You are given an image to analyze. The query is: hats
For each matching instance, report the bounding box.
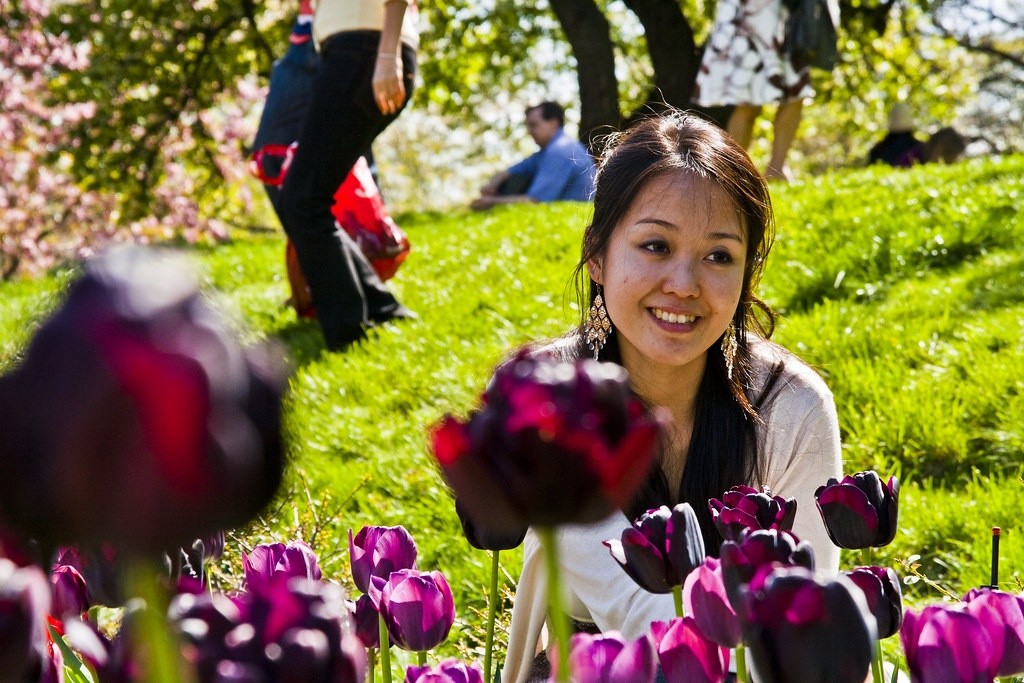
[889,104,913,131]
[289,0,314,45]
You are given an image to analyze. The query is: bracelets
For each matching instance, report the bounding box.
[377,52,401,59]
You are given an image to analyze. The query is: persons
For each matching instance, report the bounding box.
[486,111,908,682]
[691,0,811,184]
[253,0,421,352]
[466,100,597,212]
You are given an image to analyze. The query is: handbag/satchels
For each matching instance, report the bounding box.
[249,142,410,318]
[790,0,844,73]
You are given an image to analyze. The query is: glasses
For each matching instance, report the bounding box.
[529,117,550,130]
